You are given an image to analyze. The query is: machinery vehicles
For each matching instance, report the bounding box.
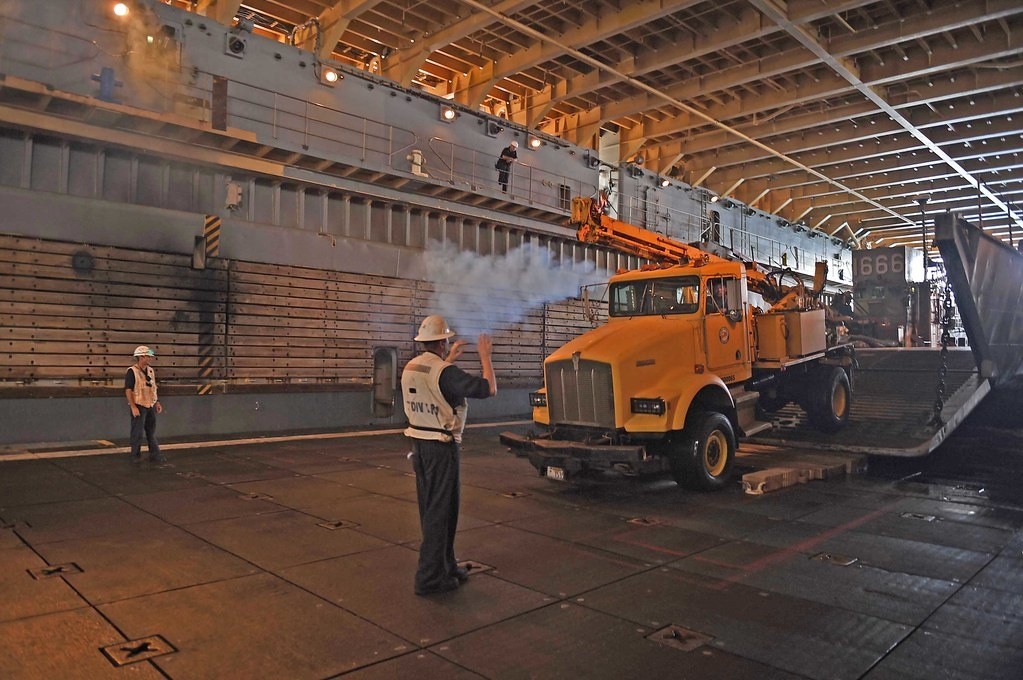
[499,173,858,493]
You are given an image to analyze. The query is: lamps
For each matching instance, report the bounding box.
[831,238,842,247]
[777,217,790,228]
[526,133,541,151]
[746,207,756,218]
[586,155,601,170]
[724,197,736,211]
[484,119,504,139]
[224,32,247,59]
[106,0,135,21]
[809,230,818,239]
[707,191,718,203]
[629,165,642,179]
[794,223,804,234]
[842,241,852,252]
[440,103,455,123]
[318,63,338,88]
[657,173,669,189]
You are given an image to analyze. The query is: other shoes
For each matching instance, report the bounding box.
[150,454,167,463]
[130,457,142,465]
[450,566,468,580]
[415,577,459,594]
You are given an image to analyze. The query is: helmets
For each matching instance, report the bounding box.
[134,346,154,356]
[511,141,518,149]
[413,315,455,342]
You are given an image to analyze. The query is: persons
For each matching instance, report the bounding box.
[401,314,497,596]
[125,345,167,465]
[496,140,518,194]
[713,284,727,310]
